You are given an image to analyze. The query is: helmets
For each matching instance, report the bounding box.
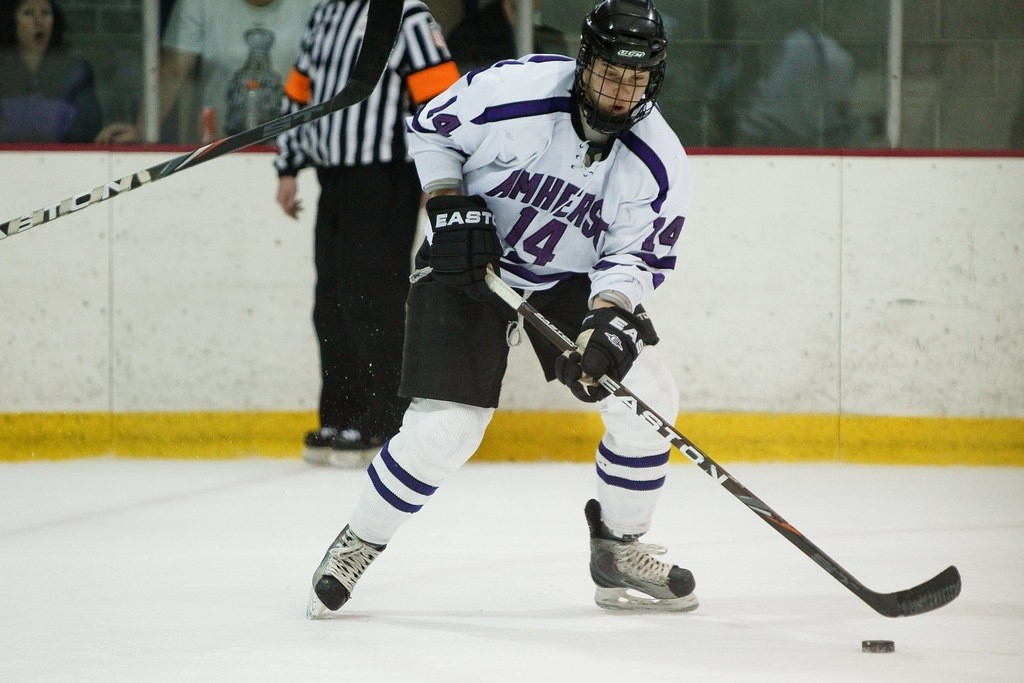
[575,0,669,135]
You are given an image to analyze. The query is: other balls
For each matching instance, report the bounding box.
[861,639,896,654]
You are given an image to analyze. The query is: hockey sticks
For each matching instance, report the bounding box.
[0,1,407,246]
[485,264,964,620]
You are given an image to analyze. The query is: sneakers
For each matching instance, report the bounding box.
[307,523,387,621]
[584,499,699,613]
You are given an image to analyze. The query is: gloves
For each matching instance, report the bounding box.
[555,303,659,404]
[426,195,503,301]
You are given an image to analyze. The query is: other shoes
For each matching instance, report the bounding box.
[303,424,384,467]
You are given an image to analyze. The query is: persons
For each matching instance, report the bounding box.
[305,0,698,621]
[275,0,460,465]
[0,0,136,143]
[444,0,857,143]
[95,0,318,147]
